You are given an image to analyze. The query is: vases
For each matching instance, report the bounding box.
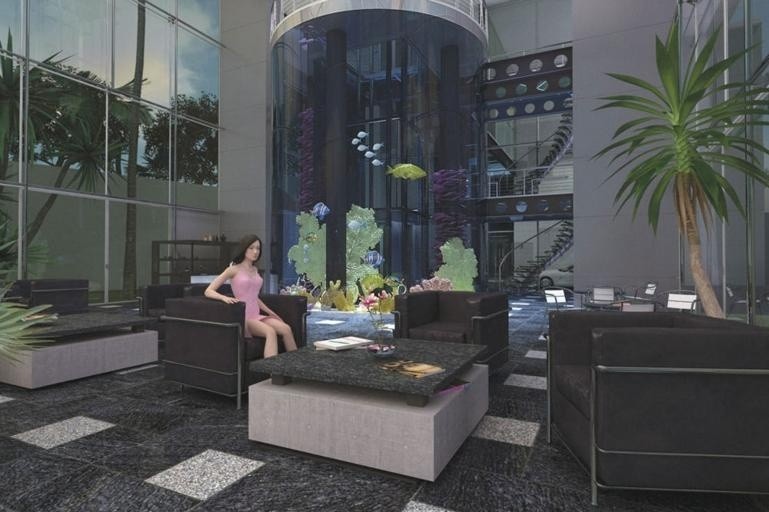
[366,320,398,357]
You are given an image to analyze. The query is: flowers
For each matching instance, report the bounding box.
[356,290,397,347]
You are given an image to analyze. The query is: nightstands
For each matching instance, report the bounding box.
[0,313,160,389]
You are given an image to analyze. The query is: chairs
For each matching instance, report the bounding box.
[546,310,768,508]
[3,279,89,315]
[542,281,700,314]
[136,283,312,410]
[392,291,510,378]
